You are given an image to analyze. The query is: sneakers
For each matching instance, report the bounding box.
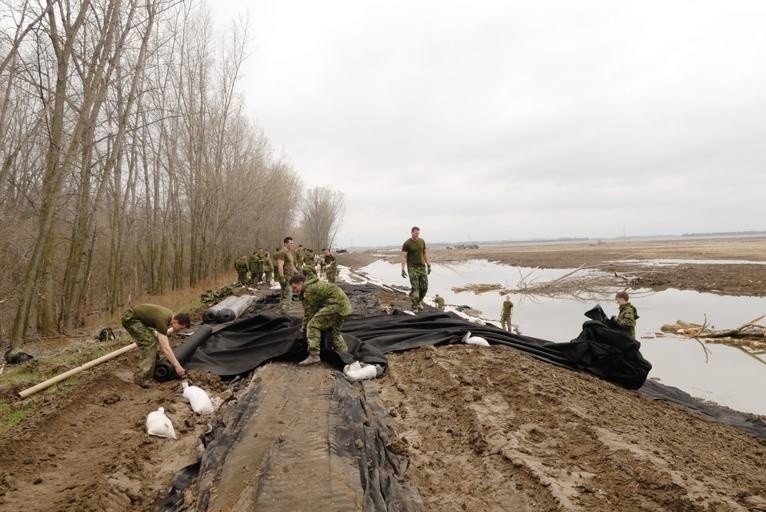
[413,304,424,314]
[137,379,160,387]
[298,354,321,367]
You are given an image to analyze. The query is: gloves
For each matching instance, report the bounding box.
[279,276,286,285]
[401,270,407,278]
[427,264,431,275]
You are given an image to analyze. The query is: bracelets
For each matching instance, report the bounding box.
[175,364,180,368]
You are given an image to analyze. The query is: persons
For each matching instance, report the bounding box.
[289,275,353,366]
[275,236,300,315]
[233,244,336,285]
[609,291,639,339]
[401,225,431,313]
[434,293,445,311]
[499,294,513,333]
[120,303,190,388]
